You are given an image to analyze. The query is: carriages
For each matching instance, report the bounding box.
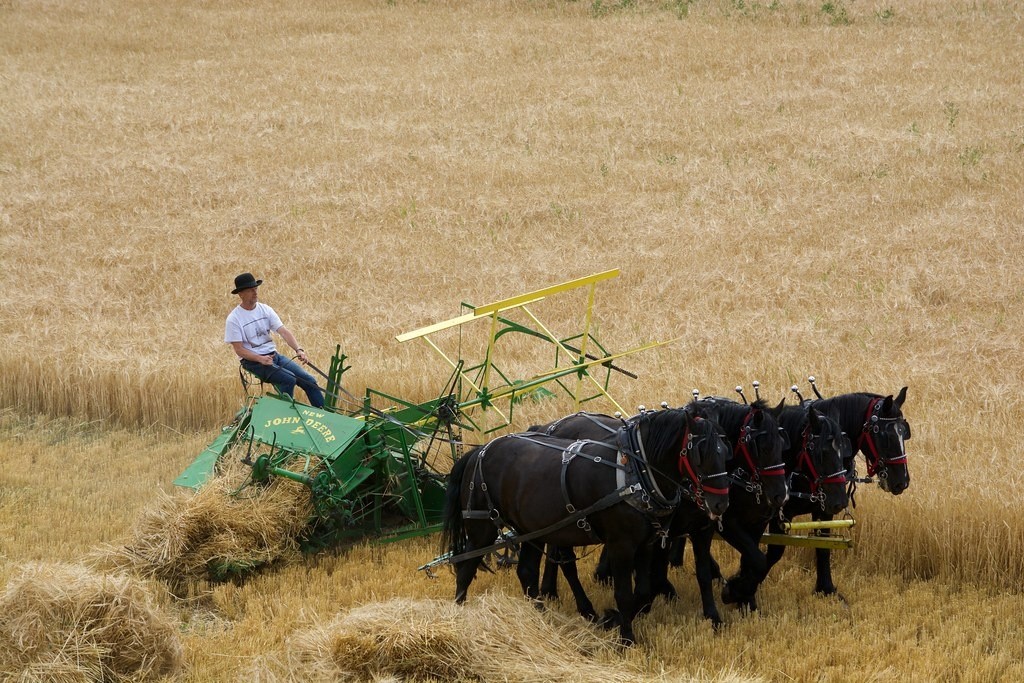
[173,268,912,651]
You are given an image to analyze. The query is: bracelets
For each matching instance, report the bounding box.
[295,348,304,353]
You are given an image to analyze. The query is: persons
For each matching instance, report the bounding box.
[225,273,325,409]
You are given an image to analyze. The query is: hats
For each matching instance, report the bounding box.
[231,273,262,294]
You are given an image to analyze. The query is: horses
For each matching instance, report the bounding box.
[437,386,912,647]
